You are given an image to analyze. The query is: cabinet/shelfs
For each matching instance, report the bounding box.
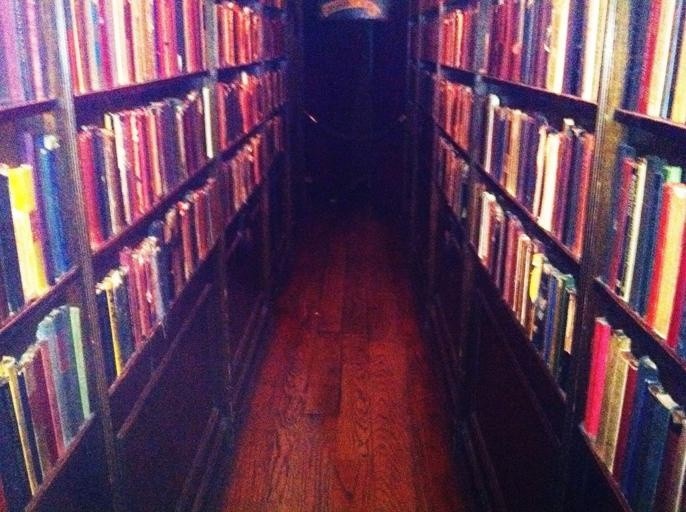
[0,0,298,512]
[405,1,686,512]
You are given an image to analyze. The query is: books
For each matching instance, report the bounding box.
[0,0,93,512]
[585,0,686,512]
[211,0,286,225]
[62,0,222,392]
[405,0,476,350]
[477,2,608,388]
